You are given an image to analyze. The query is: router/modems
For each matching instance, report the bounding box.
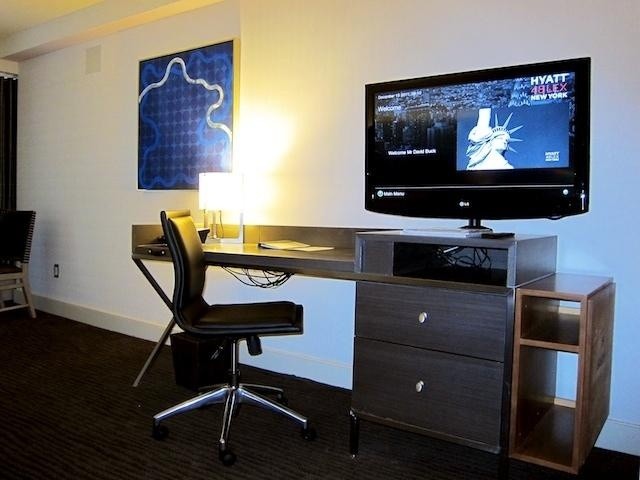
[203,209,245,244]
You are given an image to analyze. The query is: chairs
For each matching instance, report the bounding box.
[0,210,39,319]
[149,208,318,467]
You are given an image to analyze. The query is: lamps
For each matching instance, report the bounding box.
[199,171,250,242]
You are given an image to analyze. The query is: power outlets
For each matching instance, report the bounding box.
[53,263,59,278]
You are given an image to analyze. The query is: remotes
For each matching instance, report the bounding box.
[480,232,515,240]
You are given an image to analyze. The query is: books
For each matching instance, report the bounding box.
[258,239,336,253]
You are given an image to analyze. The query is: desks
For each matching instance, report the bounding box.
[132,224,403,397]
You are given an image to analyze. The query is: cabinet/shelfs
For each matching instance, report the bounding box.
[351,231,556,472]
[512,272,615,474]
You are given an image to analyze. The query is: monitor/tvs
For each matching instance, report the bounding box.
[364,56,592,237]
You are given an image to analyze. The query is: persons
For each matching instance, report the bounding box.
[464,108,516,171]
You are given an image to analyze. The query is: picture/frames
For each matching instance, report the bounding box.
[137,37,238,190]
